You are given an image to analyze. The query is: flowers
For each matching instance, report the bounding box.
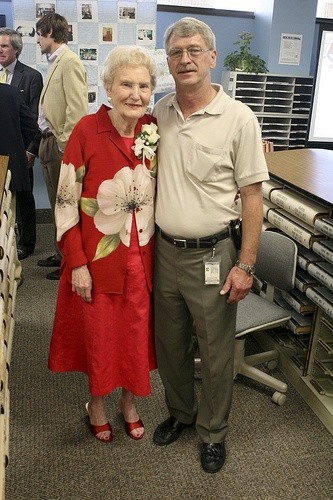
[130,122,161,173]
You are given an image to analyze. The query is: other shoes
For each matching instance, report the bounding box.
[16,245,33,260]
[202,442,226,473]
[46,269,61,280]
[37,254,61,267]
[153,416,187,446]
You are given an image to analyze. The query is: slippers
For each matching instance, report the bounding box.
[121,409,145,440]
[85,401,113,443]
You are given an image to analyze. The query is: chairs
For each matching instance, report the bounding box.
[192,231,302,409]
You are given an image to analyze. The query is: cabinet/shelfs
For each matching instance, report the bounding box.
[221,70,333,439]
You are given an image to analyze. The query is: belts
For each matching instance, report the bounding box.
[155,222,232,250]
[40,132,53,139]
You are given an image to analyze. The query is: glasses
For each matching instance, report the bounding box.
[166,46,210,58]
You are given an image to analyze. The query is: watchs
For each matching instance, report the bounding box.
[235,260,256,274]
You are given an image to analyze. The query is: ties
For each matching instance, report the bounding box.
[0,67,8,83]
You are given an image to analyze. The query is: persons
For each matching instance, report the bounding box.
[152,17,270,473]
[0,28,44,261]
[36,14,88,280]
[48,44,160,442]
